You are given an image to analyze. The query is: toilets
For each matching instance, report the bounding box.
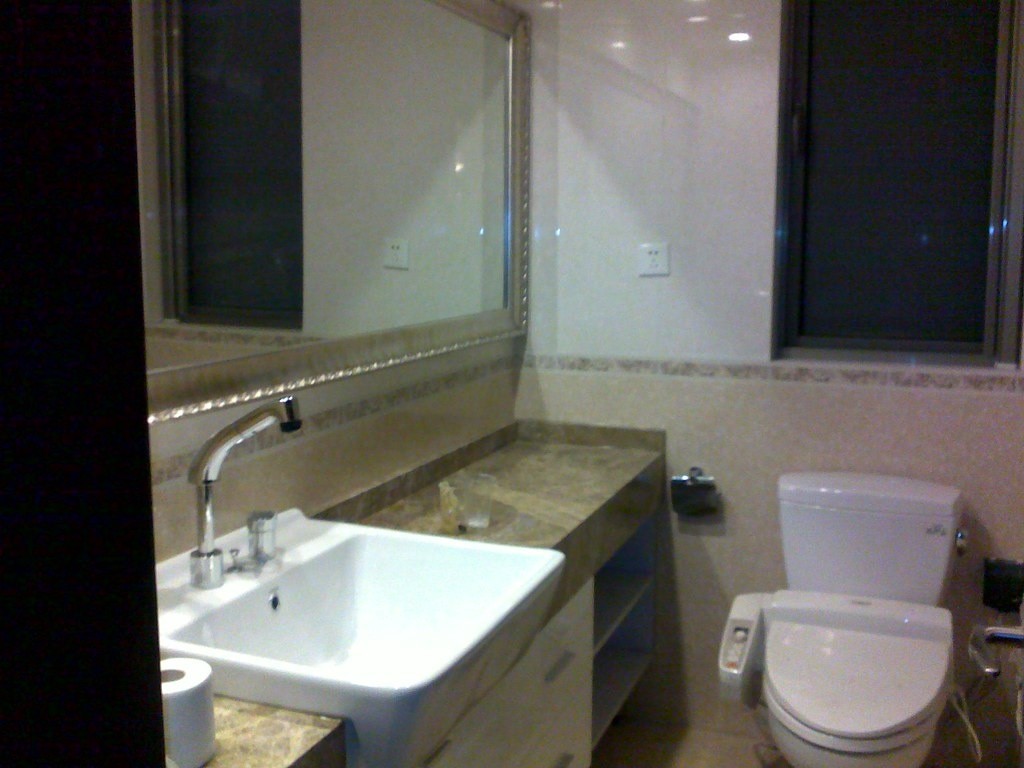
[719,470,972,768]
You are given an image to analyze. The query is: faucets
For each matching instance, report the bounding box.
[185,395,305,589]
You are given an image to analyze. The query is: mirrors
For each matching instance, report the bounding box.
[129,0,535,422]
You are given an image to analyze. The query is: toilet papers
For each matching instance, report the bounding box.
[157,656,218,768]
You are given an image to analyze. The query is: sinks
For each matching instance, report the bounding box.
[165,526,564,768]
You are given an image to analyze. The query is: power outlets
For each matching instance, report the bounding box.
[381,236,409,270]
[637,240,670,278]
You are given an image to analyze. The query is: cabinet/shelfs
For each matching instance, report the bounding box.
[593,447,657,748]
[415,573,593,768]
